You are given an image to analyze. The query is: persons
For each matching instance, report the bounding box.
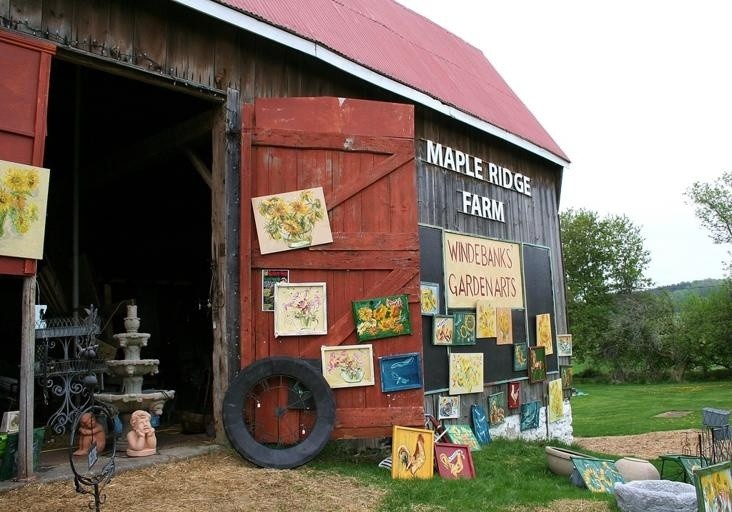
[126,410,158,456]
[73,413,106,456]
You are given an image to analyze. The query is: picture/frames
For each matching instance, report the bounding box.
[570,453,732,512]
[388,395,492,484]
[432,302,496,347]
[273,282,328,336]
[487,306,574,432]
[319,344,376,389]
[378,351,424,393]
[419,280,441,318]
